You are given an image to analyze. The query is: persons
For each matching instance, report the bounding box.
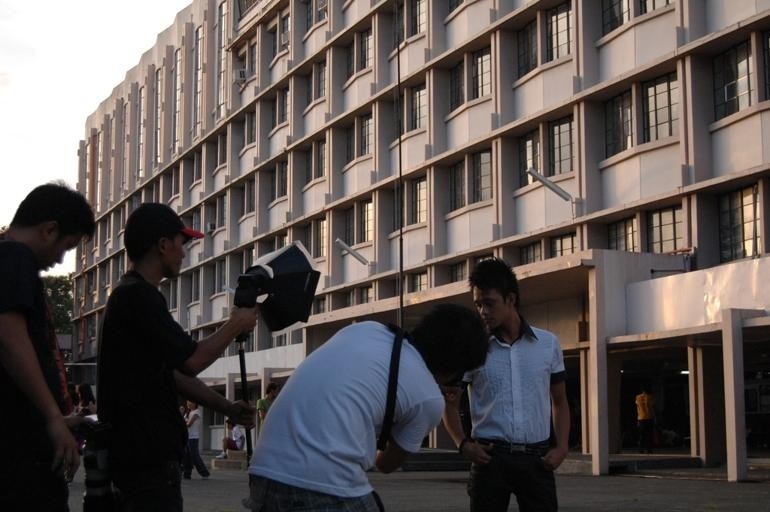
[82,200,259,511]
[78,383,96,415]
[634,384,658,454]
[67,382,79,416]
[442,257,573,512]
[257,381,284,431]
[0,181,96,511]
[216,418,245,460]
[183,400,210,480]
[241,302,493,511]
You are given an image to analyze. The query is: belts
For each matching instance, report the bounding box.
[482,442,545,454]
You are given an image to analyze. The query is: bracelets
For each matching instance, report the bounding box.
[459,436,471,453]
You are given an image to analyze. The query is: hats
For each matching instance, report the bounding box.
[129,203,205,245]
